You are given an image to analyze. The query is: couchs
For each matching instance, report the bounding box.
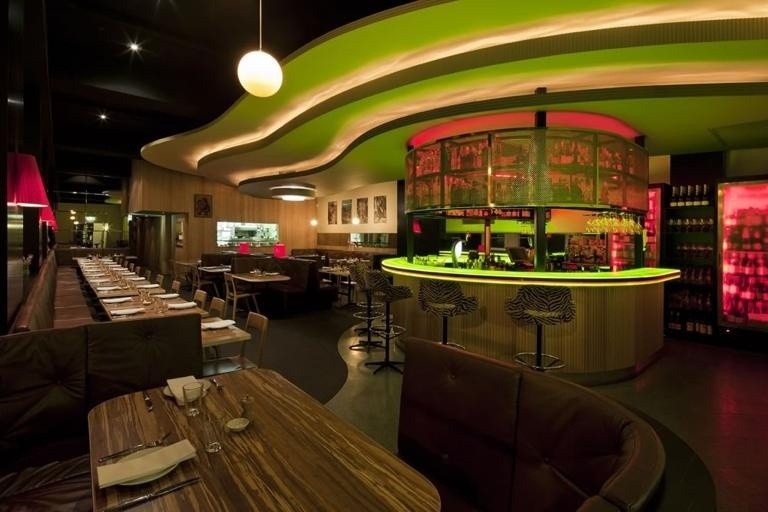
[234,256,311,313]
[390,336,720,509]
[0,244,211,475]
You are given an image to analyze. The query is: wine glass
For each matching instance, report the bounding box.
[582,212,644,235]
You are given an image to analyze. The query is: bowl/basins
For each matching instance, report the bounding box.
[226,417,250,432]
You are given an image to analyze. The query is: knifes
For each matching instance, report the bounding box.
[141,389,154,412]
[94,476,202,512]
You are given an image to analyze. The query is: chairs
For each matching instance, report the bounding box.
[362,271,413,375]
[347,264,388,354]
[290,249,376,310]
[354,288,384,338]
[502,283,577,373]
[413,277,481,351]
[3,247,269,380]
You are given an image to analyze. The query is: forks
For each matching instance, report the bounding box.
[98,431,176,464]
[207,375,225,394]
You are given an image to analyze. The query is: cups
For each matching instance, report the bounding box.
[254,267,261,276]
[200,409,225,455]
[83,254,168,315]
[182,382,204,417]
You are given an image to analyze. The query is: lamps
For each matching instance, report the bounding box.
[238,241,250,256]
[232,1,284,100]
[7,150,61,233]
[272,243,285,258]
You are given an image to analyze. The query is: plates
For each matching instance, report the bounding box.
[171,302,198,311]
[163,378,212,398]
[200,326,225,330]
[117,446,179,486]
[168,293,178,300]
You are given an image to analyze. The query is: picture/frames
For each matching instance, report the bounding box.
[193,192,214,219]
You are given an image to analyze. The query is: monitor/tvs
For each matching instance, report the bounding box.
[507,247,527,263]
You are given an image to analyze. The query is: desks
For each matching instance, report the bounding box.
[82,364,442,512]
[233,270,291,317]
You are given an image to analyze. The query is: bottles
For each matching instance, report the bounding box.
[333,263,346,272]
[238,395,255,424]
[669,243,713,282]
[666,182,712,208]
[666,218,714,232]
[669,292,714,336]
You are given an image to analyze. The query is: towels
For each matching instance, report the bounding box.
[165,373,209,406]
[90,438,204,488]
